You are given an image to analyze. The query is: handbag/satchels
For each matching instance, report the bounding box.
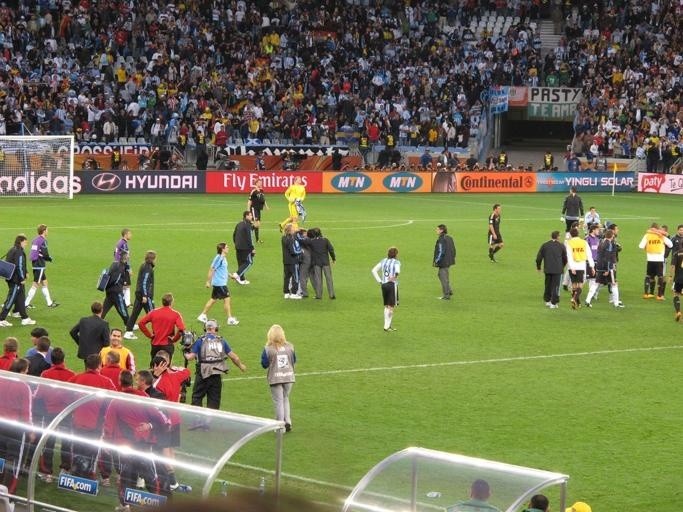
[0,247,17,281]
[96,268,110,292]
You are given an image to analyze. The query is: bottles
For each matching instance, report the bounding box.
[221,481,228,498]
[259,477,265,496]
[424,491,442,499]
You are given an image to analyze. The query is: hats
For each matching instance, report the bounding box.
[204,319,220,327]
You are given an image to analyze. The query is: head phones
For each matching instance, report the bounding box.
[204,320,218,332]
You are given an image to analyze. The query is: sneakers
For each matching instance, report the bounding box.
[233,272,250,285]
[283,284,336,300]
[37,468,193,512]
[0,301,37,328]
[561,280,626,310]
[674,311,682,322]
[48,302,59,308]
[227,317,240,326]
[383,326,398,331]
[123,323,140,340]
[197,313,209,324]
[545,300,559,309]
[641,293,667,301]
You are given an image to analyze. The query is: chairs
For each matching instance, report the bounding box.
[443,8,600,49]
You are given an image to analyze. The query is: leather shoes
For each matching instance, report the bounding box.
[441,292,453,300]
